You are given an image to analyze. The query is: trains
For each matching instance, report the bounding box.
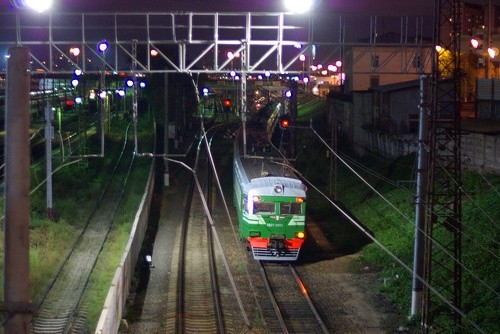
[231,101,307,263]
